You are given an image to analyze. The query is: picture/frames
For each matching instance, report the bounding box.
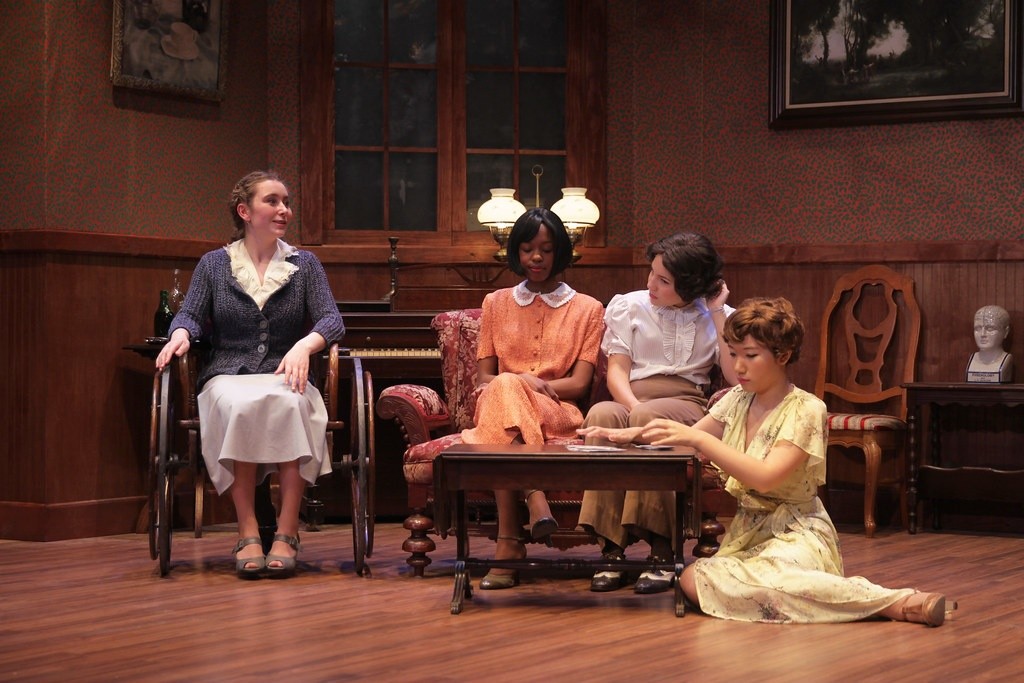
[109,0,230,105]
[769,0,1024,133]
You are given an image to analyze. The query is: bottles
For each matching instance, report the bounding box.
[154,290,174,339]
[169,269,186,314]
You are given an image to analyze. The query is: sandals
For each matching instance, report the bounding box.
[232,531,304,580]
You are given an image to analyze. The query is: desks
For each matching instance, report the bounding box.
[894,369,1024,533]
[434,441,693,617]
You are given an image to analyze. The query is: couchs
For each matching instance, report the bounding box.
[376,307,726,577]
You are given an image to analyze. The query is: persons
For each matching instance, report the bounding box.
[155,170,346,579]
[462,206,605,588]
[576,232,737,594]
[964,304,1014,385]
[576,296,958,626]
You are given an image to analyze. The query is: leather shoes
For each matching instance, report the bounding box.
[634,554,676,593]
[591,548,626,589]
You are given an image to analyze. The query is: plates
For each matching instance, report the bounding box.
[144,336,168,345]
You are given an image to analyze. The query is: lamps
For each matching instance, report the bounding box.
[477,187,527,263]
[550,188,599,260]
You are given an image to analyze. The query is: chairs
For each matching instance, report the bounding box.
[813,264,923,526]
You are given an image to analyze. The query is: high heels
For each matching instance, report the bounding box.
[904,586,958,627]
[525,488,559,546]
[479,535,528,589]
